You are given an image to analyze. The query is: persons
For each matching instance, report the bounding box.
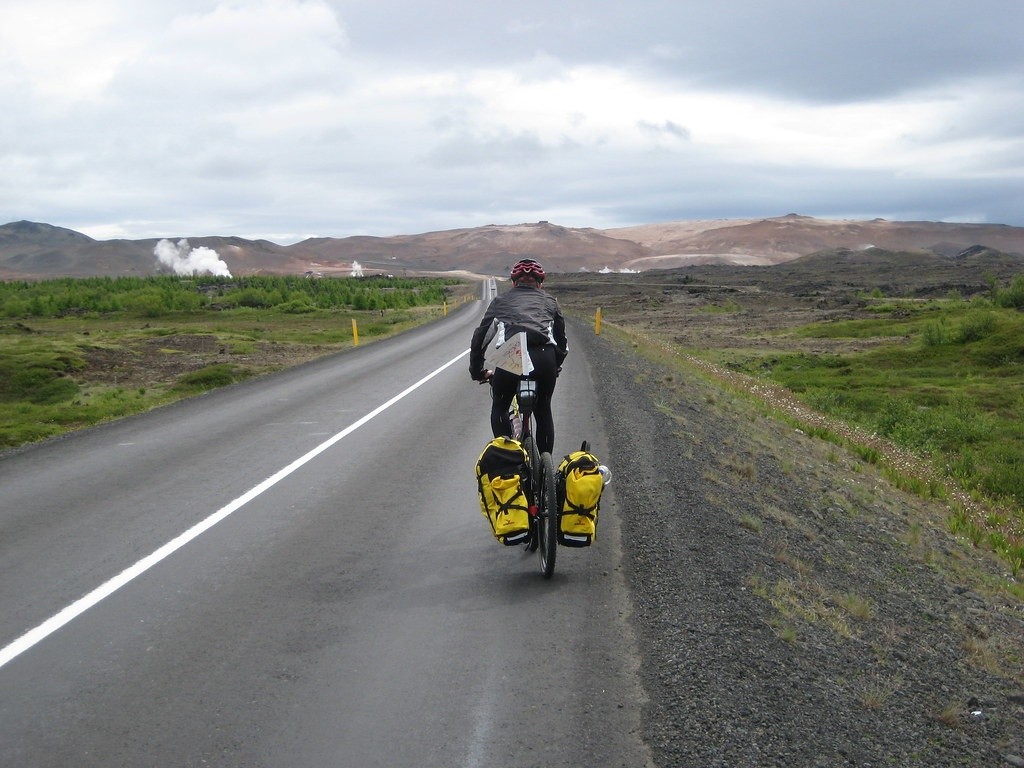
[468,259,568,457]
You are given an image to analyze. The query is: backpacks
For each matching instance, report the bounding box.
[556,440,607,549]
[477,438,533,544]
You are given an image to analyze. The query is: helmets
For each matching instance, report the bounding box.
[510,258,545,286]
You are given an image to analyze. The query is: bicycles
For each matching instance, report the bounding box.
[478,367,565,576]
